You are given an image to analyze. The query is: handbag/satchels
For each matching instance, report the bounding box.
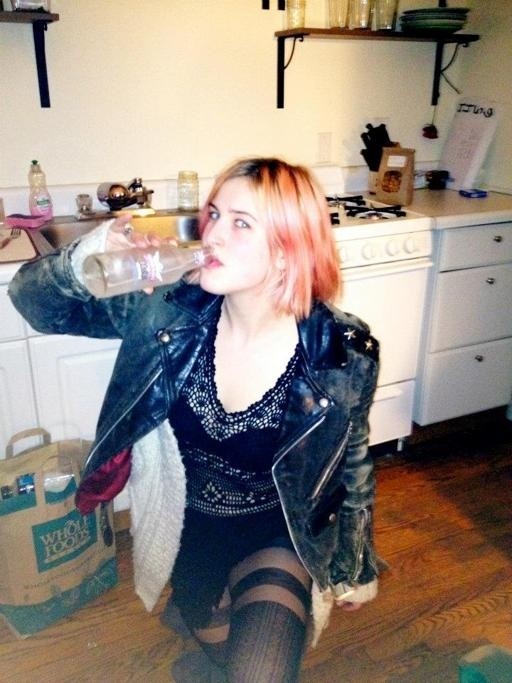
[1,427,119,641]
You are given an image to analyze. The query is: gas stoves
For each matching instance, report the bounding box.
[321,191,430,229]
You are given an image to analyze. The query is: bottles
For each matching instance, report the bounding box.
[81,246,212,299]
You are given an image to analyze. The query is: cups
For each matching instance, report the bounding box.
[328,0,399,30]
[177,169,200,212]
[287,0,306,30]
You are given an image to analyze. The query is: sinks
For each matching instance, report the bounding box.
[33,214,202,252]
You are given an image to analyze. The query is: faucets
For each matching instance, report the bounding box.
[99,184,148,214]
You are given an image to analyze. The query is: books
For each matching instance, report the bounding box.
[438,96,499,190]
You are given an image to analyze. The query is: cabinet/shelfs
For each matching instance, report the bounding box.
[0,262,42,464]
[411,218,512,428]
[24,262,138,532]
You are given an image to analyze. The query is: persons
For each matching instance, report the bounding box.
[7,156,380,682]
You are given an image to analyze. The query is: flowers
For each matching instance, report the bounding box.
[422,94,438,138]
